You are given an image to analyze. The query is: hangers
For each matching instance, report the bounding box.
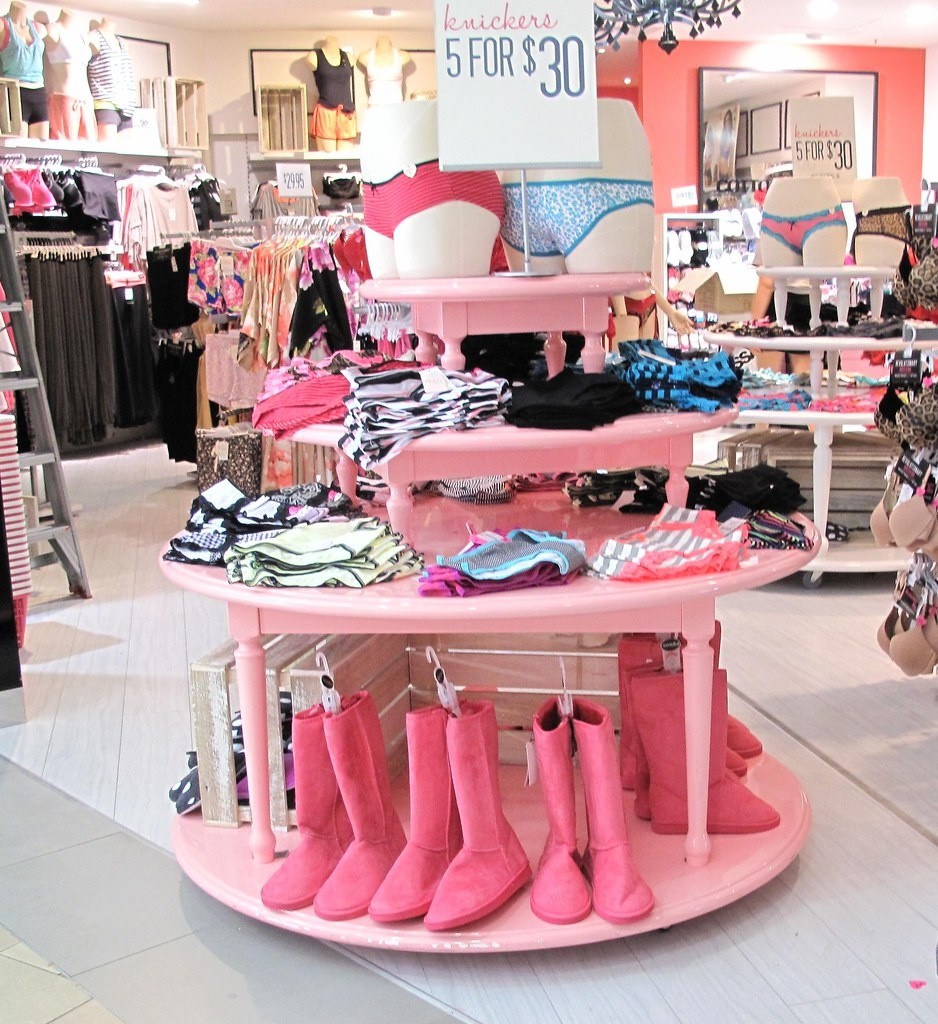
[886,317,932,371]
[268,163,361,187]
[2,150,99,169]
[12,230,101,260]
[115,164,226,191]
[910,177,936,213]
[148,214,364,257]
[351,303,413,342]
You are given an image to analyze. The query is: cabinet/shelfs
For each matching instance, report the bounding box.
[652,212,745,464]
[135,274,812,953]
[706,267,938,589]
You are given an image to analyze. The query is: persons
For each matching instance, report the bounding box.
[760,175,848,268]
[307,36,356,152]
[46,9,94,141]
[0,0,49,139]
[612,283,694,354]
[853,176,911,268]
[501,99,655,274]
[751,279,814,374]
[88,18,134,143]
[359,37,412,106]
[360,99,504,279]
[718,110,735,181]
[705,128,713,186]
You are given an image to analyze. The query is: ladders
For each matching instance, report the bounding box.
[0,192,92,600]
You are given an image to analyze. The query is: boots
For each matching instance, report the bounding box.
[261,696,356,911]
[368,696,464,922]
[619,619,762,821]
[312,690,407,921]
[631,669,781,834]
[422,699,532,931]
[569,698,654,924]
[533,695,593,925]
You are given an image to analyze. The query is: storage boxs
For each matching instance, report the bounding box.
[0,76,23,137]
[187,625,413,839]
[406,634,624,763]
[255,84,310,154]
[716,424,905,533]
[671,267,758,314]
[140,77,210,153]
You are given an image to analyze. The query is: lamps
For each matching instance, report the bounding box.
[594,0,741,52]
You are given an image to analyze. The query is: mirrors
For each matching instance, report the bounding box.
[697,64,880,212]
